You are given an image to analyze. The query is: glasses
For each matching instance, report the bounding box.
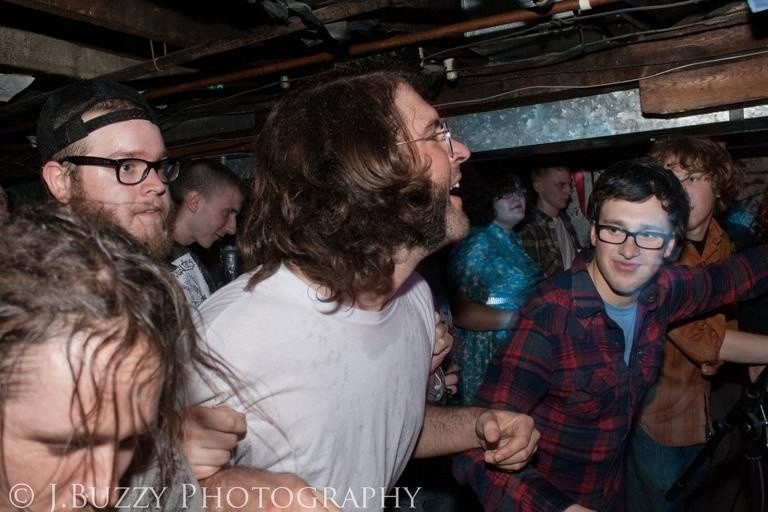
[393,117,454,159]
[57,153,183,187]
[594,217,678,252]
[497,184,530,200]
